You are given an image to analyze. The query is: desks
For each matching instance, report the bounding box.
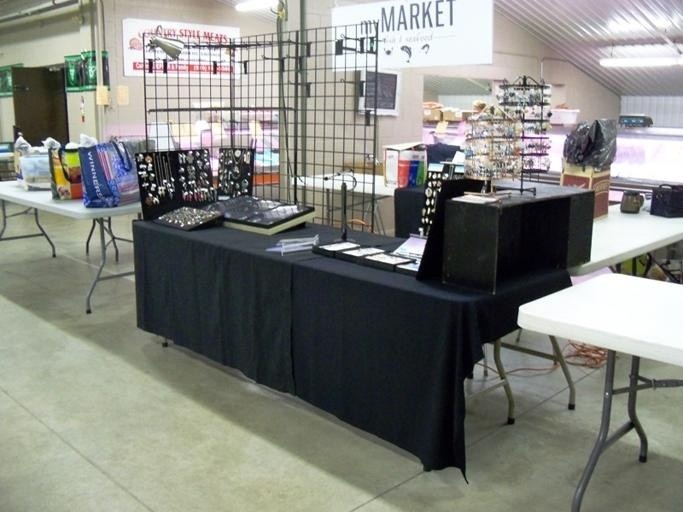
[0,181,143,314]
[568,199,683,277]
[133,210,574,482]
[516,274,682,511]
[292,172,395,238]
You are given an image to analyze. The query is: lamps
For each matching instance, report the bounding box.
[148,35,185,59]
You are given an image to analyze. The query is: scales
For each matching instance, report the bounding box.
[619,115,653,127]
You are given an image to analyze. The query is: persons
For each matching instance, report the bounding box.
[472,100,486,112]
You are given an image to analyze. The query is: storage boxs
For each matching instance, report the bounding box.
[382,143,427,188]
[422,101,579,123]
[560,158,610,218]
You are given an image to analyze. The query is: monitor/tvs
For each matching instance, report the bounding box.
[358,68,402,117]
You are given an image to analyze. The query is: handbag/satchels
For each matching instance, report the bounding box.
[48,145,84,200]
[79,140,155,207]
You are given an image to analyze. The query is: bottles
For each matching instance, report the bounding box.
[399,155,420,188]
